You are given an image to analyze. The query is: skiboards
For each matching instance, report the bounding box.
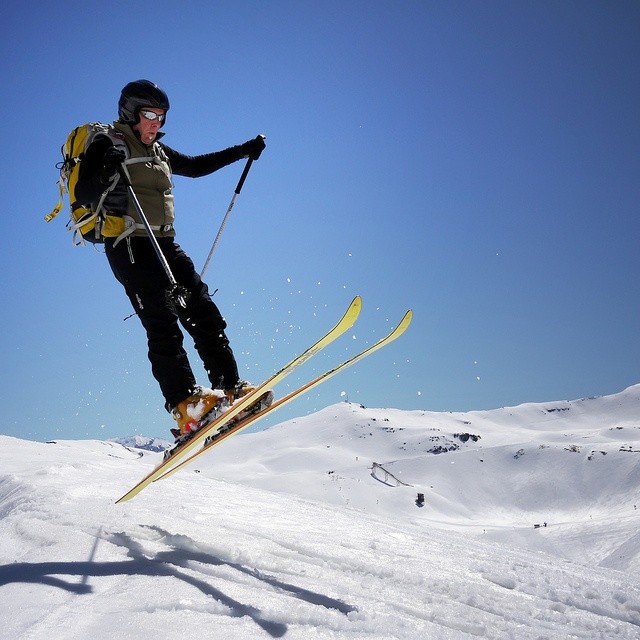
[113,295,413,504]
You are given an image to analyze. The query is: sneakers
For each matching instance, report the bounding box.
[216,385,272,432]
[175,390,232,437]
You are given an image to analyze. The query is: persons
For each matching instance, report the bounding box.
[74,80,272,446]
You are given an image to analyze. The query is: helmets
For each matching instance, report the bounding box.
[119,80,169,125]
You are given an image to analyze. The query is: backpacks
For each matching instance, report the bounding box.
[44,122,119,244]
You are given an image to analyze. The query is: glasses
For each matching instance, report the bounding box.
[139,110,165,121]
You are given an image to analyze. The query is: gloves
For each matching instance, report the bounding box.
[101,147,124,177]
[244,137,266,159]
